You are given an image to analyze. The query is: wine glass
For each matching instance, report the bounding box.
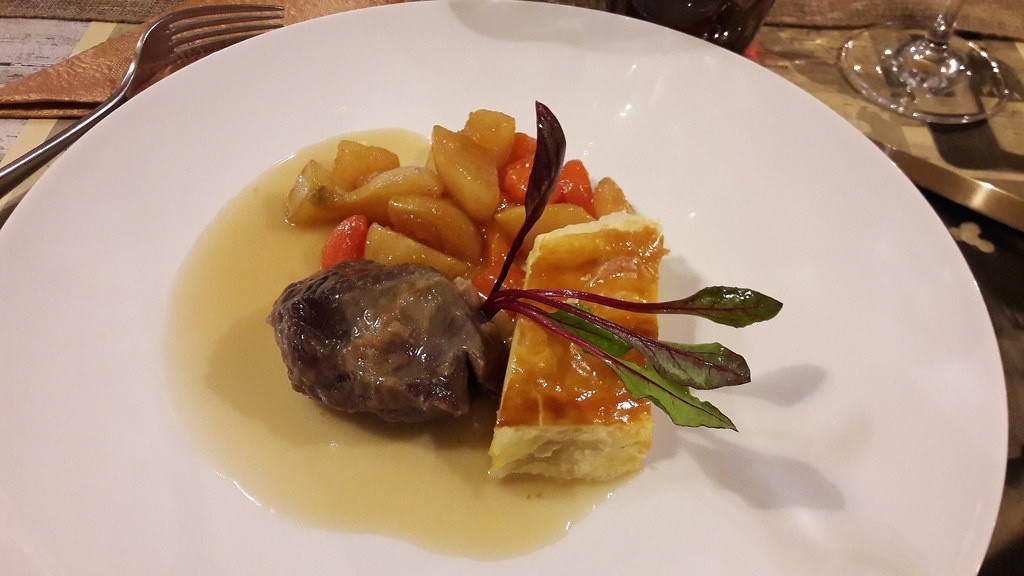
[836,0,1014,129]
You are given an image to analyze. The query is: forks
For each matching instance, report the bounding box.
[1,3,285,203]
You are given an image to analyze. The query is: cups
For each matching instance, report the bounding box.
[602,1,775,58]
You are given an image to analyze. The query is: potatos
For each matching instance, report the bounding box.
[282,104,637,327]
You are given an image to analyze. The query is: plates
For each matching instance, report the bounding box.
[0,0,1012,574]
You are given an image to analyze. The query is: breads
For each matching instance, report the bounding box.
[486,210,670,478]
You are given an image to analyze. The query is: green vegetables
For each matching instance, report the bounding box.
[477,99,783,432]
